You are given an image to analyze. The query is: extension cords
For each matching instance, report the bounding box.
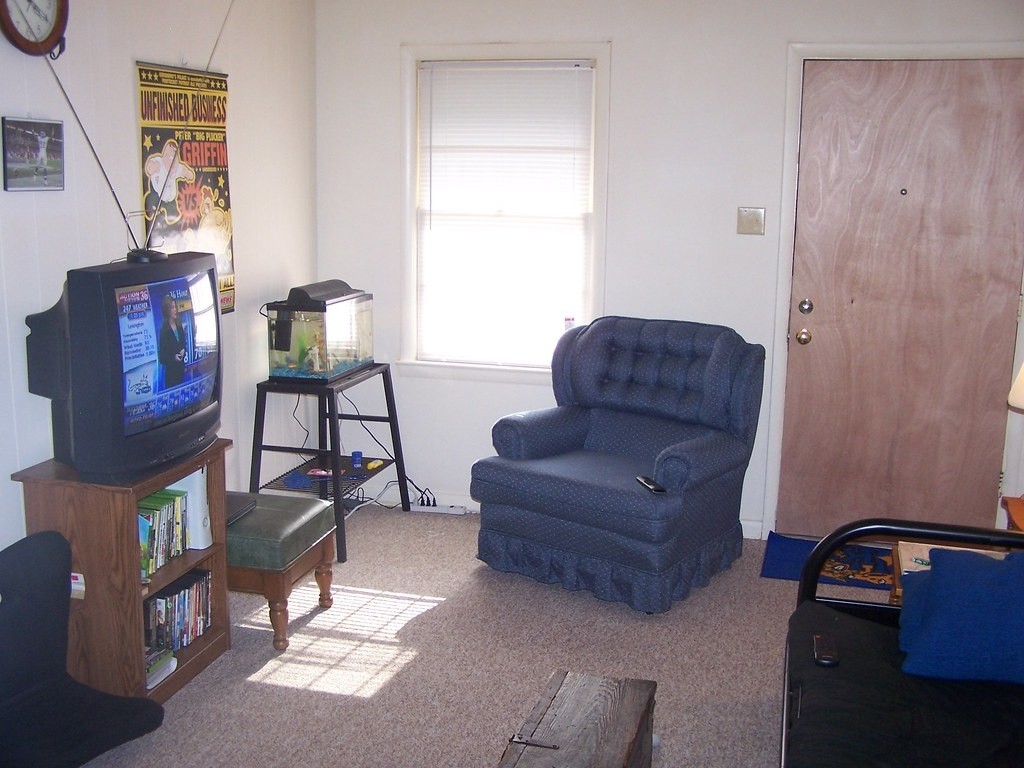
[410,503,467,515]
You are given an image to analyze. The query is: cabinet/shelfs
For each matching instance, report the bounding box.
[250,364,411,562]
[10,438,234,703]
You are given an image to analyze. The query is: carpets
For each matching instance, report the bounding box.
[761,530,894,590]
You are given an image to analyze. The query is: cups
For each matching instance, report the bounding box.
[352,451,362,468]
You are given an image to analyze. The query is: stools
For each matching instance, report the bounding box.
[225,491,337,649]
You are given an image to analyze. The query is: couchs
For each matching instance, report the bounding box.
[470,316,765,613]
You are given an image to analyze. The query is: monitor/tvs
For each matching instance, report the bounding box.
[26,251,224,475]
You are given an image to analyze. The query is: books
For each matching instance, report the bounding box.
[137,488,190,578]
[143,568,212,689]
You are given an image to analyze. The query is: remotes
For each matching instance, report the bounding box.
[812,632,841,666]
[636,475,666,494]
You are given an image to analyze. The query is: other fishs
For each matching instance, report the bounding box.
[271,309,373,373]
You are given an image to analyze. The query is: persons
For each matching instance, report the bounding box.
[158,295,186,390]
[32,127,55,186]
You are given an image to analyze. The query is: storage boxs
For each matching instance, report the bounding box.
[497,669,658,767]
[259,280,373,384]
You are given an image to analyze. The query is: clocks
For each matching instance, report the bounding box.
[0,0,69,60]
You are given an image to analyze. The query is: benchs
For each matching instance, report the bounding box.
[780,518,1023,768]
